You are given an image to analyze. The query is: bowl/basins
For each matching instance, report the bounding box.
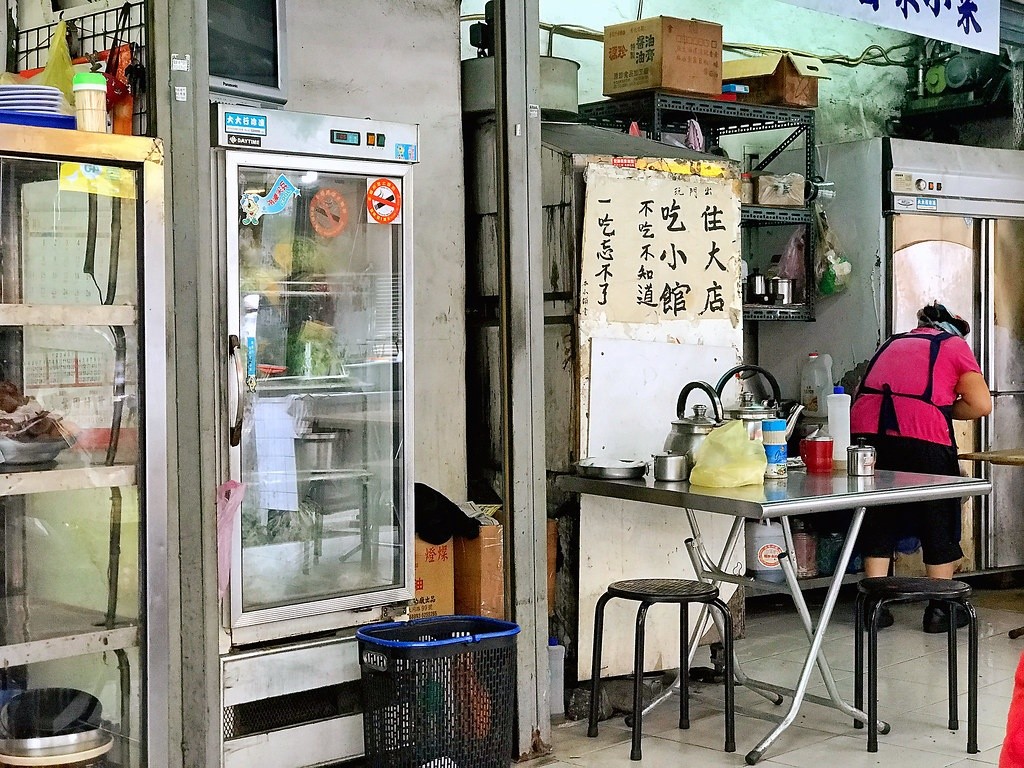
[0,435,76,462]
[294,426,348,476]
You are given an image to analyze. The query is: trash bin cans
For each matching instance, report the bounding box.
[356,615,521,768]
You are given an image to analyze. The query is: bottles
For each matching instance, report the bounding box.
[547,635,564,715]
[240,294,259,376]
[745,517,786,570]
[799,353,833,418]
[827,386,851,460]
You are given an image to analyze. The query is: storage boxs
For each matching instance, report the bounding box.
[722,52,832,109]
[602,15,723,98]
[382,534,454,622]
[453,525,504,620]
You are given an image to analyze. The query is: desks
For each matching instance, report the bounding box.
[958,448,1024,640]
[550,459,992,766]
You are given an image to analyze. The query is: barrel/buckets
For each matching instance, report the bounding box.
[0,688,114,768]
[792,519,818,577]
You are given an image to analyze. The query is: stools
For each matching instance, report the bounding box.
[854,577,978,754]
[587,579,735,761]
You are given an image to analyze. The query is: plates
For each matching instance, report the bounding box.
[0,85,64,114]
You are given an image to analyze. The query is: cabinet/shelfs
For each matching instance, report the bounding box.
[577,91,817,321]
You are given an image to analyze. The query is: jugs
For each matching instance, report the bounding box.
[663,381,724,478]
[716,365,805,441]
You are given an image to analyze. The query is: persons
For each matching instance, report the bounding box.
[850,302,994,631]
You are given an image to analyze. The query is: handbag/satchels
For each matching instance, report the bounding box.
[690,419,767,487]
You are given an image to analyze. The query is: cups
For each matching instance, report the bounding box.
[800,437,834,475]
[763,442,787,479]
[771,278,798,303]
[772,278,793,304]
[742,280,748,302]
[746,267,765,295]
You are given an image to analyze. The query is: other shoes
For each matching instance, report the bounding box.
[864,604,894,630]
[924,606,970,633]
